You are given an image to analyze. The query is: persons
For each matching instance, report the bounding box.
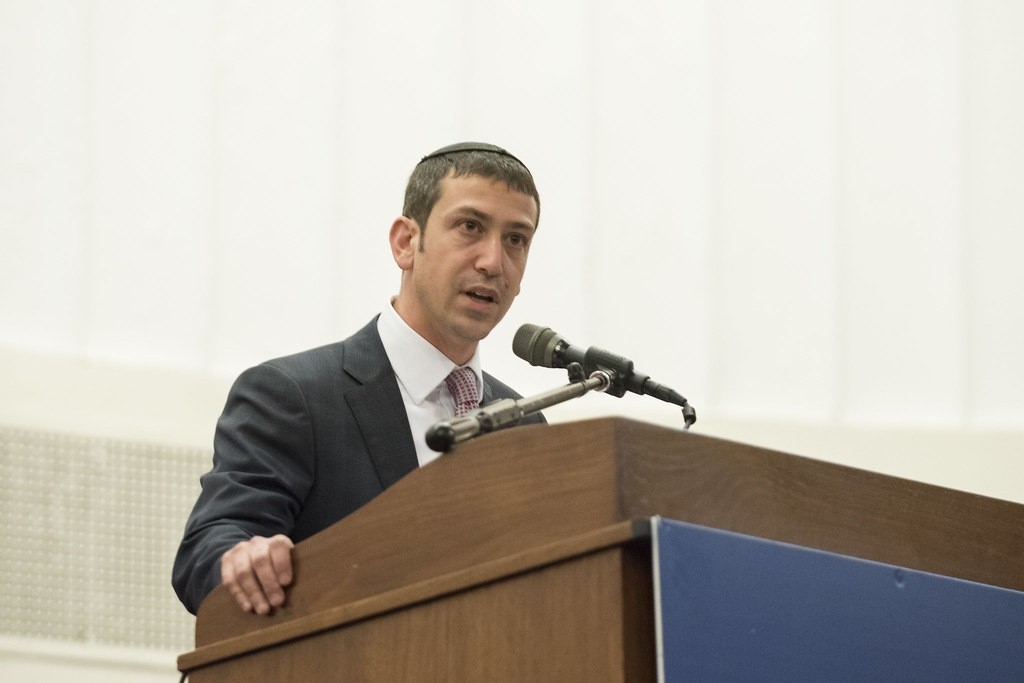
[170,141,549,616]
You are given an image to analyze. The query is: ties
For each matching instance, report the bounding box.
[445,368,479,418]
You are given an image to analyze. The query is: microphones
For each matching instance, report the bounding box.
[512,323,687,406]
